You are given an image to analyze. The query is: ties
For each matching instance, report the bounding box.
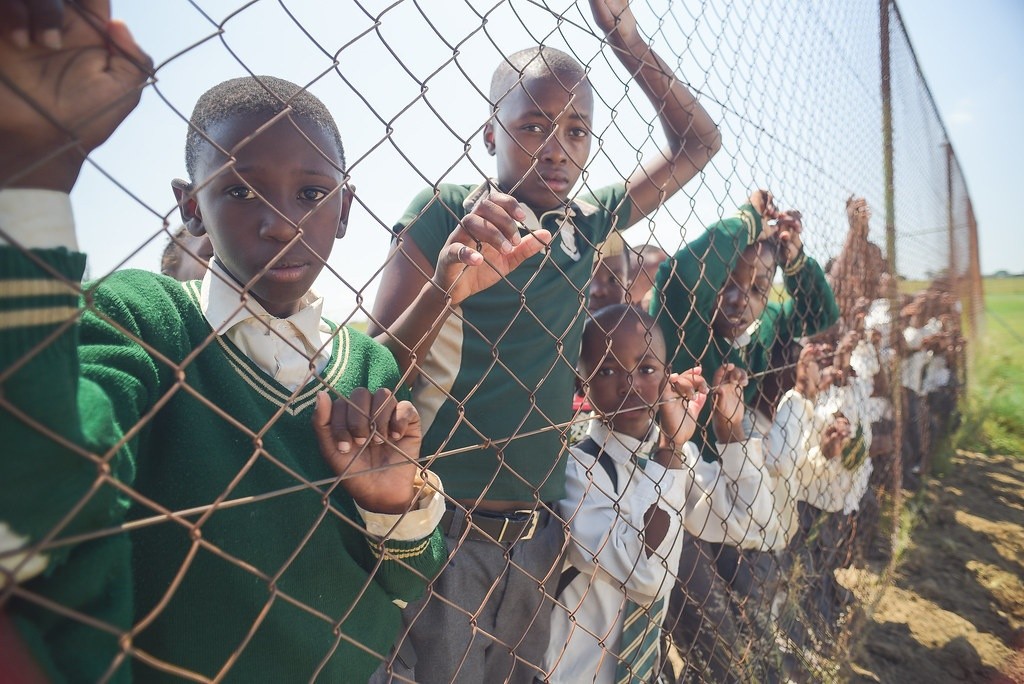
[615,443,673,684]
[841,412,869,471]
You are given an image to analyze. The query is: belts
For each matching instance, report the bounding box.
[440,503,552,543]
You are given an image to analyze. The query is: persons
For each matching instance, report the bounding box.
[160,0,968,684]
[0,0,446,684]
[368,1,723,684]
